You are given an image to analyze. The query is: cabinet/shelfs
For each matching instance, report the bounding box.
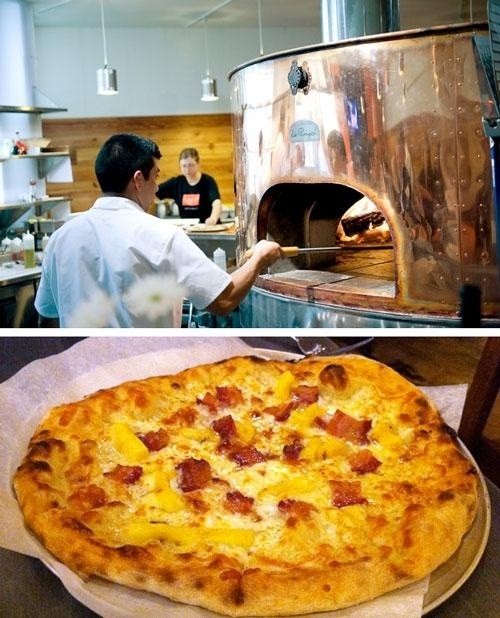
[0,107,72,212]
[0,262,60,328]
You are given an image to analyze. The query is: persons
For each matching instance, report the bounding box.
[33,131,286,328]
[390,132,439,237]
[150,146,225,226]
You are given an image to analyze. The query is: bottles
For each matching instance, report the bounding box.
[1,236,22,262]
[213,246,227,273]
[41,232,49,251]
[22,229,35,268]
[13,131,21,154]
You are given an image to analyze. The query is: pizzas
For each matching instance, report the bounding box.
[8,353,479,617]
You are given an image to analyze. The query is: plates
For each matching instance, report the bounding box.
[421,432,492,615]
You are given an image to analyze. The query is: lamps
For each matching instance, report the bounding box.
[201,18,219,101]
[97,1,117,96]
